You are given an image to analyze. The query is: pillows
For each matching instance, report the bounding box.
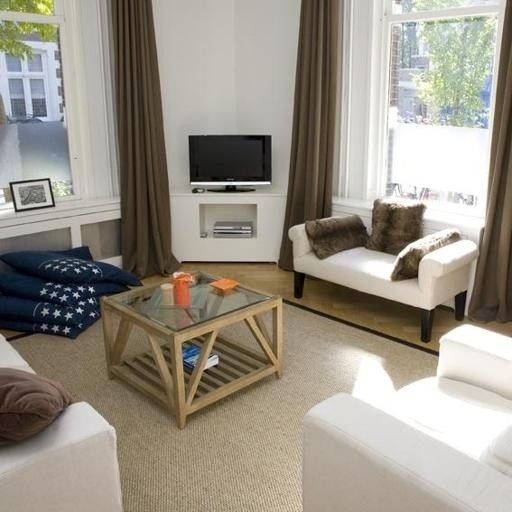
[0,366,75,445]
[366,198,426,254]
[302,214,369,260]
[0,245,146,340]
[384,226,461,283]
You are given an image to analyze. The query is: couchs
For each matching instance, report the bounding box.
[0,320,130,512]
[284,193,481,344]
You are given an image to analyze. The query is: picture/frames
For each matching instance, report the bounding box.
[10,177,56,213]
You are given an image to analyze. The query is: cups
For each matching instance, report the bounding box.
[172,272,192,308]
[162,284,174,306]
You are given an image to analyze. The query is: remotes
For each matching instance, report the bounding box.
[192,188,199,193]
[197,189,205,192]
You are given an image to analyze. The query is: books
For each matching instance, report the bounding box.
[182,345,219,371]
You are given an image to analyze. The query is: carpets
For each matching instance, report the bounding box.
[6,275,442,512]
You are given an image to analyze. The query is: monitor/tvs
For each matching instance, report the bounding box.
[189,134,272,193]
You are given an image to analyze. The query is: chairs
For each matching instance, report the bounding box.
[293,317,512,511]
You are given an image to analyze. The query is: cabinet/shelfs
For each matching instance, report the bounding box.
[167,188,293,268]
